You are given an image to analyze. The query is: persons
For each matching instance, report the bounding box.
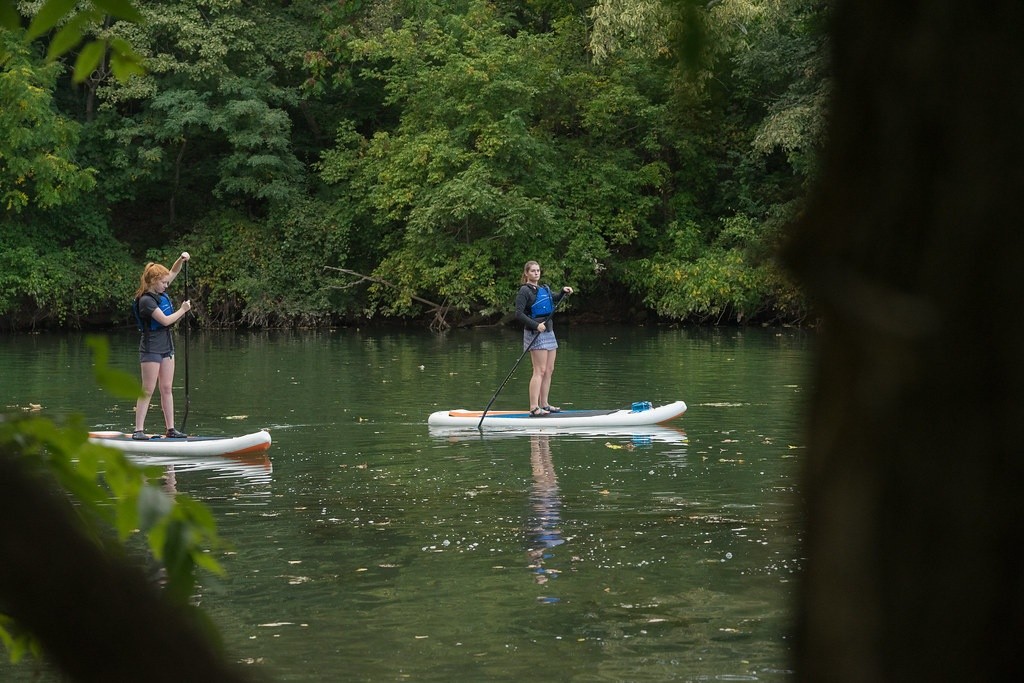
[132,252,191,440]
[515,261,573,417]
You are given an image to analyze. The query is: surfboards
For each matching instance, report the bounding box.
[86,428,273,457]
[427,401,688,429]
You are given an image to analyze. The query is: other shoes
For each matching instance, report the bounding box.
[166,428,187,438]
[132,430,149,440]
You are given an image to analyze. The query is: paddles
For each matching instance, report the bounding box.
[476,290,569,429]
[176,258,191,434]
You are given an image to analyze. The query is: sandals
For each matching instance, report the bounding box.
[529,407,551,417]
[542,405,560,413]
